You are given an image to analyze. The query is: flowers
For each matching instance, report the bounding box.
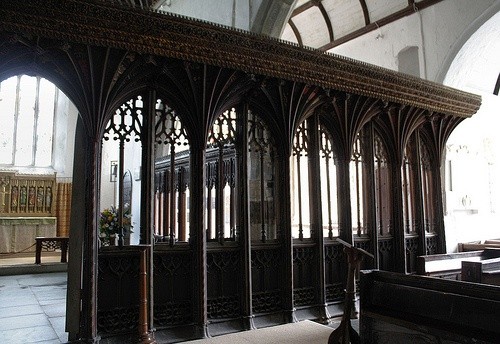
[100,204,136,239]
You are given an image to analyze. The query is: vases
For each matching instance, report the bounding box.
[100,236,117,249]
